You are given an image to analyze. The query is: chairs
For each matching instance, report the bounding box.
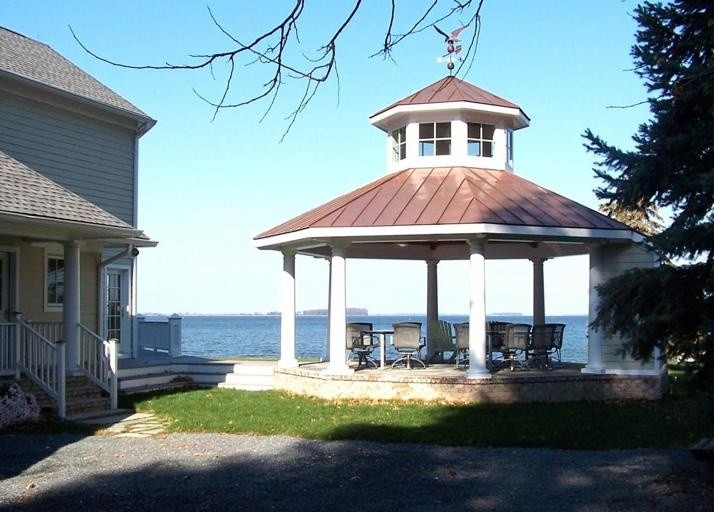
[344,319,566,372]
